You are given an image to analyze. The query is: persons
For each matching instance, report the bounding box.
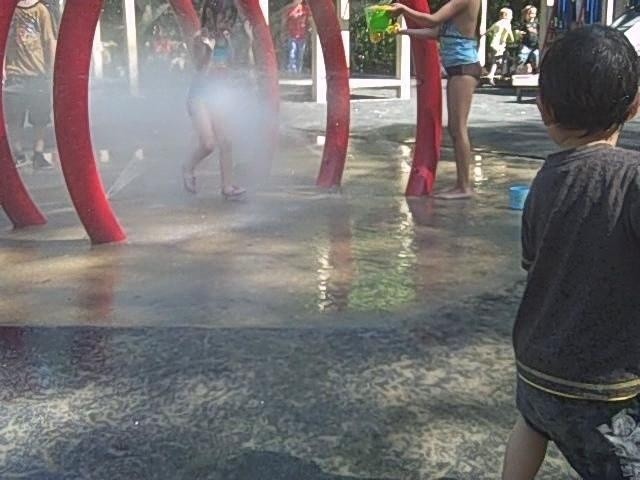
[182,0,248,197]
[384,0,484,200]
[480,7,515,86]
[2,0,54,168]
[27,1,235,78]
[279,1,312,80]
[501,22,640,479]
[513,3,541,73]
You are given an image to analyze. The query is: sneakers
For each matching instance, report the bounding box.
[221,183,246,196]
[13,150,33,167]
[181,161,198,193]
[33,152,53,170]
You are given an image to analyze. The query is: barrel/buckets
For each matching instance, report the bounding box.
[509,186,530,209]
[364,6,394,32]
